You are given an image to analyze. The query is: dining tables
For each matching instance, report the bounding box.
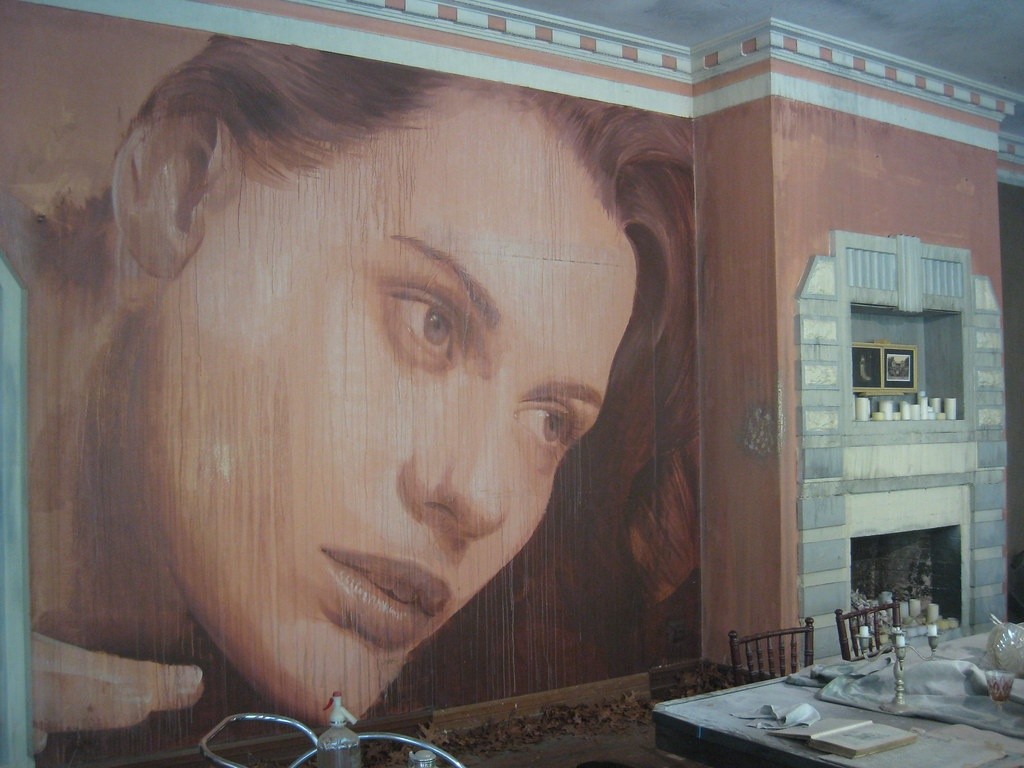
[652,620,1024,767]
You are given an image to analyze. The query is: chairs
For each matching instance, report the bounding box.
[728,617,814,686]
[834,596,901,661]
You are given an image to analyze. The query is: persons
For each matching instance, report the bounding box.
[27,2,704,768]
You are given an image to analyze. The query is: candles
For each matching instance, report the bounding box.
[856,390,957,420]
[859,592,960,649]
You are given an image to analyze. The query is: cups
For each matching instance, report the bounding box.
[985,670,1015,712]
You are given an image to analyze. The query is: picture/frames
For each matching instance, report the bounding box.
[852,342,918,396]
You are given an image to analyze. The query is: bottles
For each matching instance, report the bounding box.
[315,691,363,768]
